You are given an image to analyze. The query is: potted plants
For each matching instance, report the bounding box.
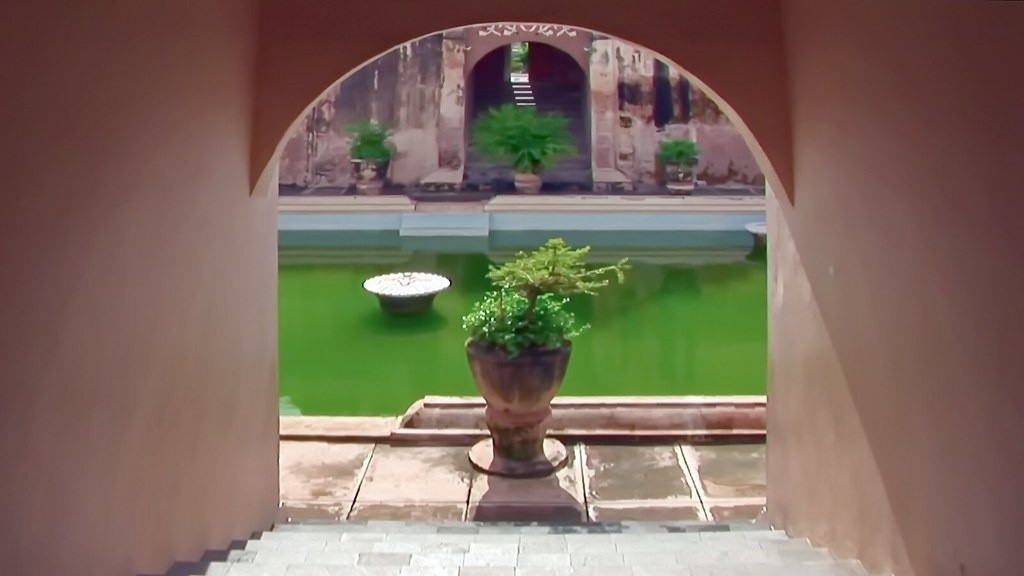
[658,140,699,183]
[343,121,398,191]
[462,237,636,478]
[471,101,578,194]
